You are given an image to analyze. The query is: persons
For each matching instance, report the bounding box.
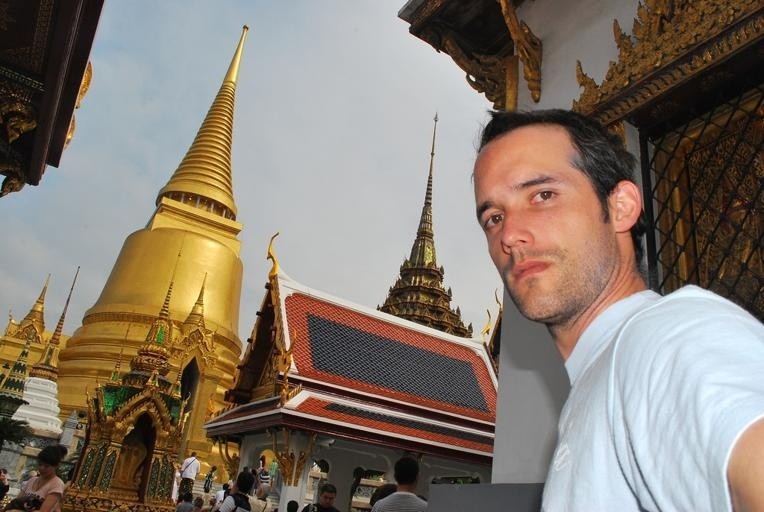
[65,467,75,485]
[4,443,68,512]
[22,471,37,491]
[0,467,10,503]
[177,448,428,512]
[470,107,764,510]
